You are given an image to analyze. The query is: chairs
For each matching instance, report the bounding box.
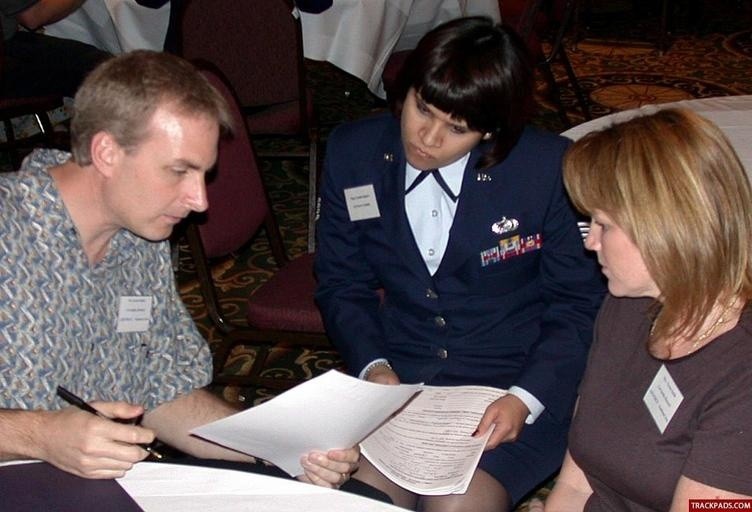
[181,57,386,386]
[499,0,590,129]
[0,30,115,173]
[179,1,321,254]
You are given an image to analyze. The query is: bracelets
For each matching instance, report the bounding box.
[364,362,393,382]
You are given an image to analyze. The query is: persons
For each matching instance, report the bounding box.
[525,106,752,512]
[316,16,610,512]
[0,47,395,512]
[0,1,118,99]
[133,1,334,14]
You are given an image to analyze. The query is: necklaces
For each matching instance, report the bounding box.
[650,292,740,356]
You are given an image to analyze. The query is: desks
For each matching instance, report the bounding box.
[0,458,414,512]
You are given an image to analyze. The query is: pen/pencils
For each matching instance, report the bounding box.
[57,385,162,459]
